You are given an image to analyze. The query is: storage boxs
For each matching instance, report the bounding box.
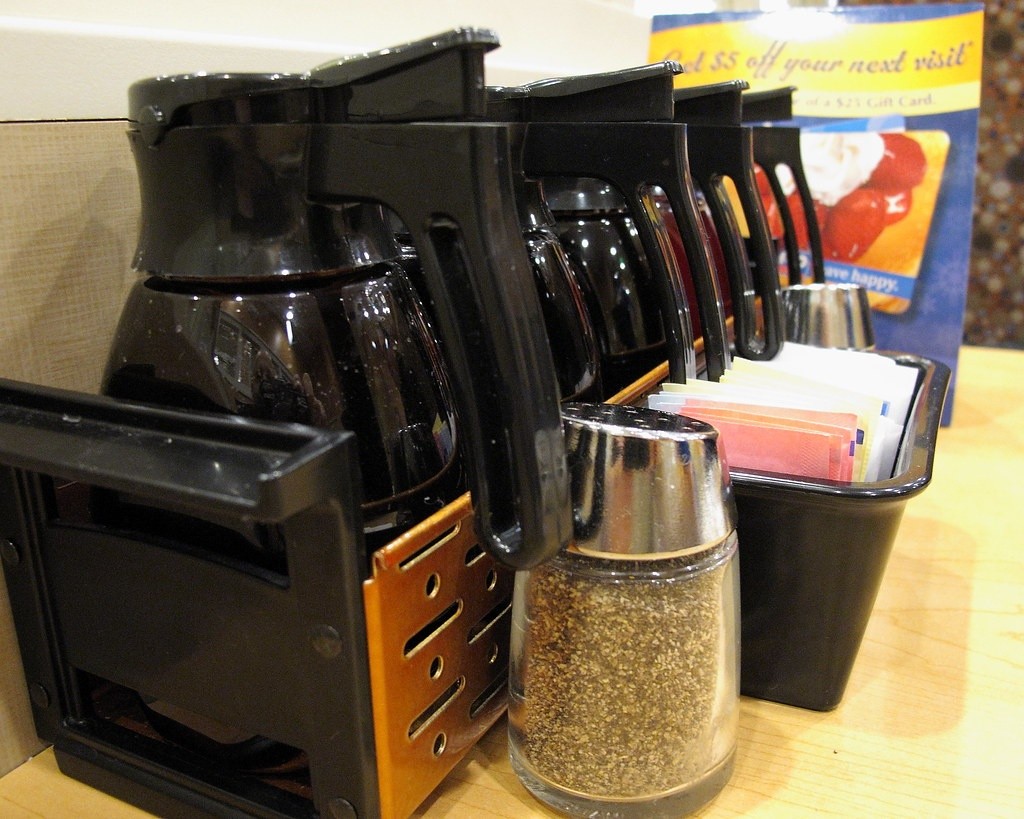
[696,341,952,715]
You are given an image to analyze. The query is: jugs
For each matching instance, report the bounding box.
[100,27,825,579]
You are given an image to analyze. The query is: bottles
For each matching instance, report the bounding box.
[505,401,742,819]
[780,283,876,353]
